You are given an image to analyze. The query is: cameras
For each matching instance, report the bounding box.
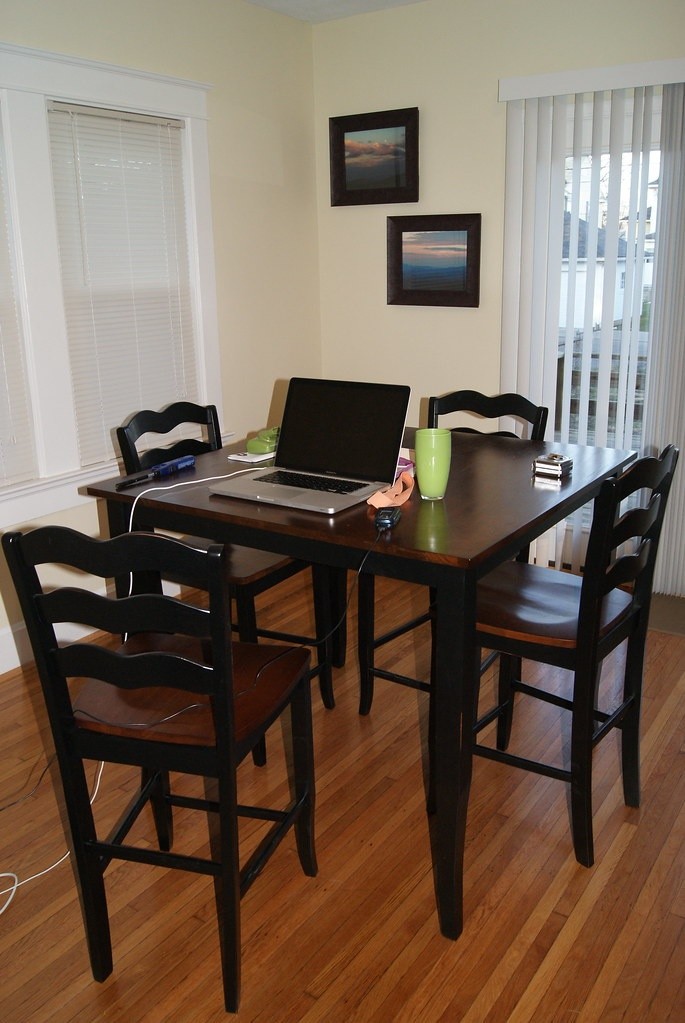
[532,455,573,477]
[531,474,572,493]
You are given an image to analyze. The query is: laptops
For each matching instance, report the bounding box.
[208,377,411,513]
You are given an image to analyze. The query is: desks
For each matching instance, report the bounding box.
[87,431,638,941]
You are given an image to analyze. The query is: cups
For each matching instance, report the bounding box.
[416,500,448,553]
[415,429,452,501]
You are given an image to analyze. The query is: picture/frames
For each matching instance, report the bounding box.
[386,213,482,308]
[328,107,419,207]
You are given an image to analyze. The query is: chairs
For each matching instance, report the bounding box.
[359,391,549,750]
[1,526,316,1013]
[471,445,679,867]
[116,401,336,711]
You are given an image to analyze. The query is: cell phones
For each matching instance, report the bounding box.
[374,506,401,528]
